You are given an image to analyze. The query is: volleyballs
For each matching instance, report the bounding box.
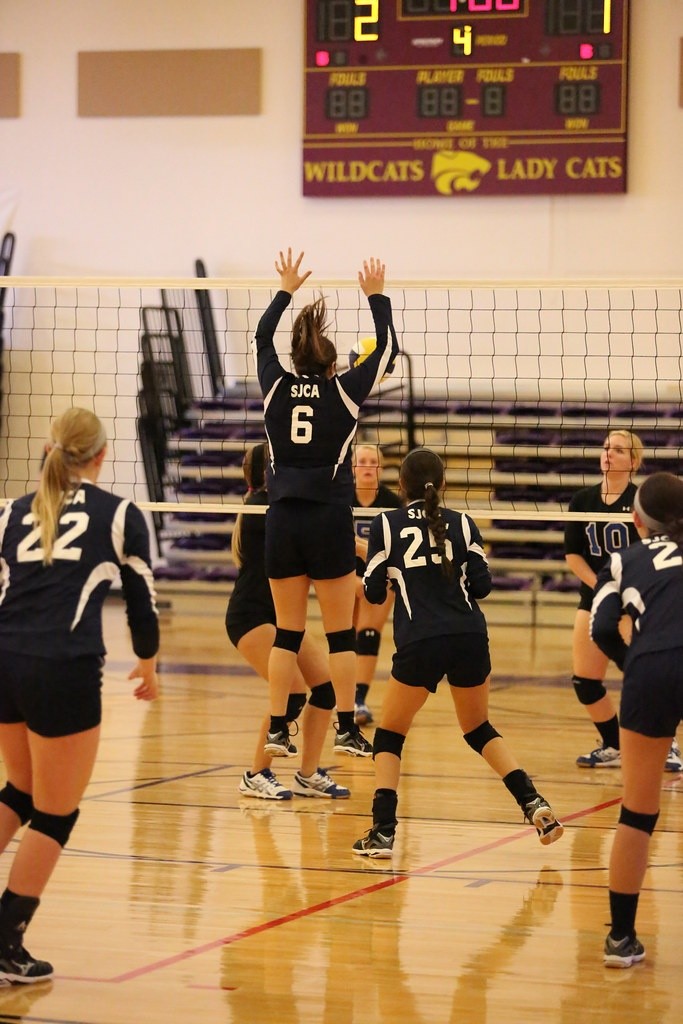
[348,336,397,384]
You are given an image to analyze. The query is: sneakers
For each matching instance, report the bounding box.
[577,739,621,768]
[353,829,394,859]
[1,946,54,983]
[603,933,645,967]
[264,729,298,758]
[354,705,373,724]
[664,741,683,772]
[523,796,563,845]
[292,768,351,799]
[239,768,293,800]
[333,722,374,757]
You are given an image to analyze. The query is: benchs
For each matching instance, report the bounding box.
[166,409,683,592]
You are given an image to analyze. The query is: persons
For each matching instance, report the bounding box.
[589,471,683,968]
[352,448,564,859]
[0,407,161,984]
[564,431,683,771]
[226,444,350,801]
[253,248,399,759]
[352,442,403,724]
